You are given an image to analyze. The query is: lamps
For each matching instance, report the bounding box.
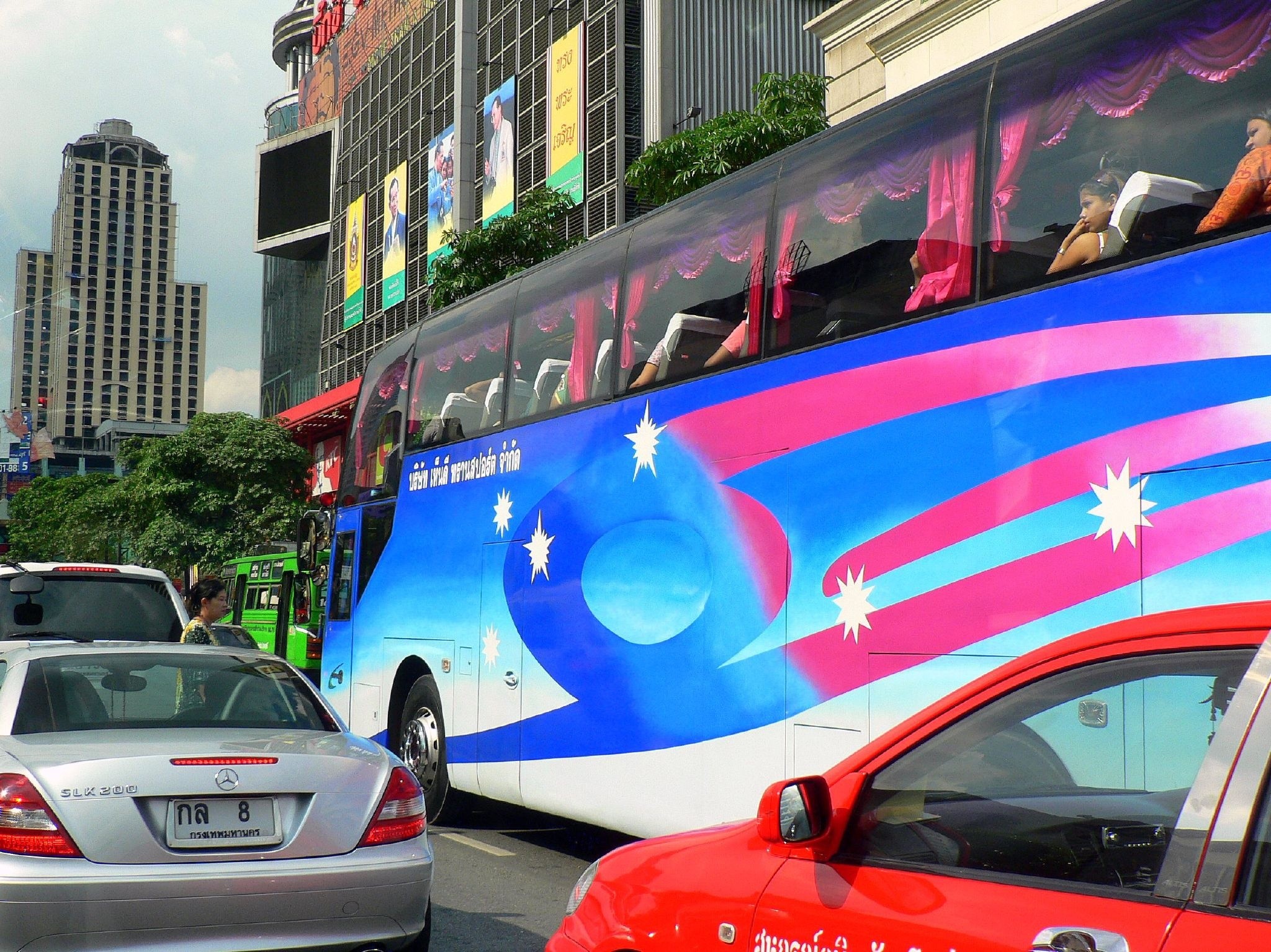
[673,106,701,130]
[335,343,354,350]
[424,110,445,116]
[283,0,362,65]
[374,324,390,331]
[482,61,504,67]
[381,149,400,153]
[548,7,571,15]
[343,180,360,185]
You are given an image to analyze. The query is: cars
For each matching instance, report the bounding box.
[0,646,434,952]
[543,599,1271,951]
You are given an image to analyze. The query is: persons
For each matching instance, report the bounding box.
[484,95,514,194]
[383,178,405,260]
[422,287,749,448]
[1192,101,1271,236]
[428,134,453,226]
[1044,166,1132,276]
[173,575,228,718]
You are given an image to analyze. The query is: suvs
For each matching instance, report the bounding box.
[0,559,191,723]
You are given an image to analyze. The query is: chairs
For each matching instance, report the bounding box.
[438,171,1218,438]
[221,676,292,722]
[59,671,110,723]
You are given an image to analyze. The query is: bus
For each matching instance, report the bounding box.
[210,623,258,649]
[214,549,353,670]
[299,0,1271,844]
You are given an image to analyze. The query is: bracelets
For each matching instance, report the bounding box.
[1057,247,1065,256]
